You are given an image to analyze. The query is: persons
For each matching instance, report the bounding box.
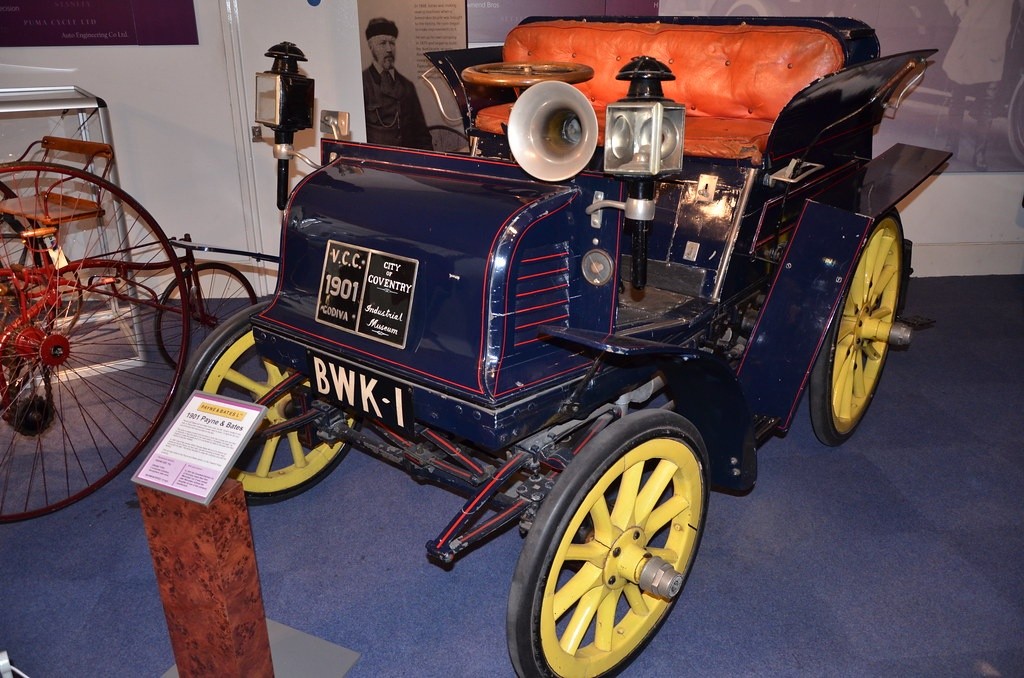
[363,18,435,151]
[939,0,1024,172]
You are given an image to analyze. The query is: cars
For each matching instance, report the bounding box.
[709,0,1024,168]
[172,9,957,677]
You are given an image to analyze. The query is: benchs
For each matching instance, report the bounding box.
[474,16,879,170]
